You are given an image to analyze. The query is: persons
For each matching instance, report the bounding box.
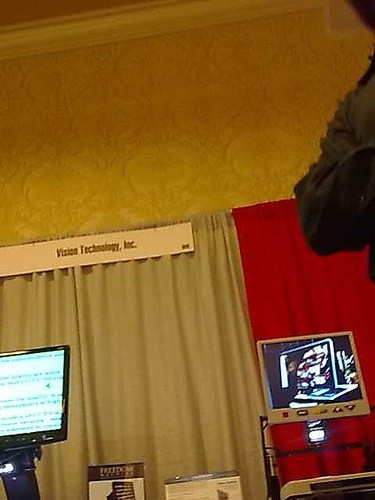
[292,0,375,284]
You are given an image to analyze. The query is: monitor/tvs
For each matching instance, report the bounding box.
[0,345,71,449]
[256,331,372,423]
